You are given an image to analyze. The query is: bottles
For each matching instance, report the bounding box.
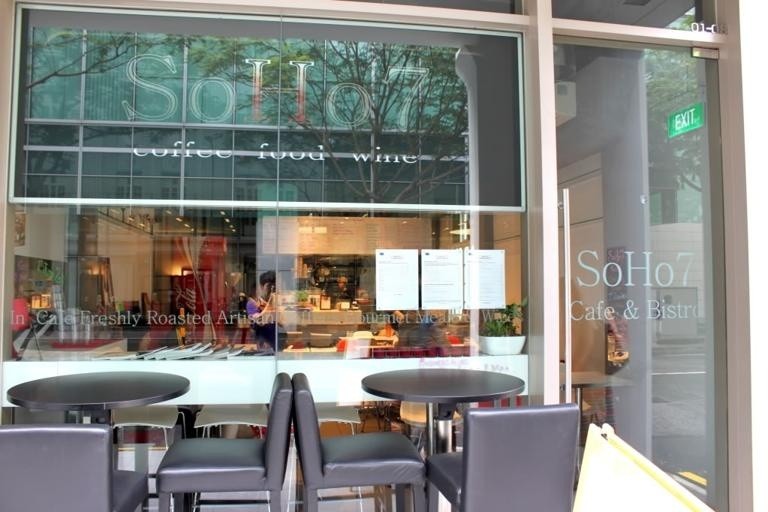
[151,292,161,316]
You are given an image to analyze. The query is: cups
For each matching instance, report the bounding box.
[386,324,392,337]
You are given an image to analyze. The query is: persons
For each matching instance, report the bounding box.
[385,309,445,347]
[245,271,288,353]
[592,307,628,437]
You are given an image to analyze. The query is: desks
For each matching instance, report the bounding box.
[7,371,190,424]
[560,371,637,479]
[362,369,525,456]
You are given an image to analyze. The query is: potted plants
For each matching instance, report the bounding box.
[479,296,528,356]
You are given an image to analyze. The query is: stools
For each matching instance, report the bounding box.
[110,406,187,451]
[194,405,268,438]
[316,406,362,435]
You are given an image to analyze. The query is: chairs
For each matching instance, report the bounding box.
[293,373,425,512]
[156,374,296,512]
[425,403,582,512]
[0,423,149,512]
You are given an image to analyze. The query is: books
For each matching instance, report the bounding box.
[92,343,276,361]
